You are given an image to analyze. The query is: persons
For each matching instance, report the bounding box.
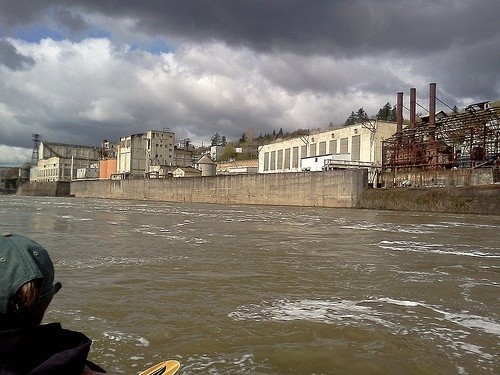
[0,230,95,375]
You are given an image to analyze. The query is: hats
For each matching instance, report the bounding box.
[0,232,63,315]
